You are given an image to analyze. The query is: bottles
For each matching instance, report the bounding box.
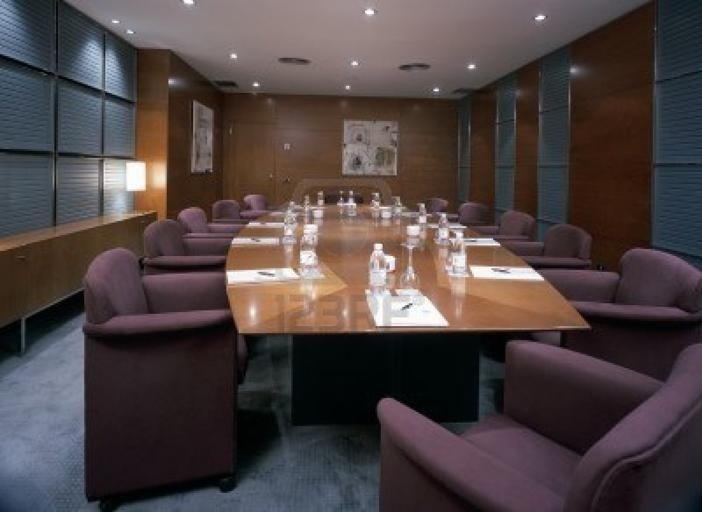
[370,191,403,218]
[336,190,356,209]
[386,255,396,272]
[282,190,326,267]
[368,241,388,294]
[454,233,466,273]
[419,203,426,225]
[439,214,449,240]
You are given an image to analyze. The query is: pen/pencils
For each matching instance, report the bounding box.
[261,222,266,223]
[252,237,260,242]
[258,272,275,275]
[464,239,483,242]
[402,303,413,310]
[492,268,510,273]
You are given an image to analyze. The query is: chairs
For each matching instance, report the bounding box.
[497,223,594,270]
[319,192,364,205]
[81,247,248,510]
[378,338,700,511]
[417,192,537,242]
[142,193,269,272]
[535,247,701,382]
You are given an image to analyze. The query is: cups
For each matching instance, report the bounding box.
[399,243,416,285]
[407,226,418,236]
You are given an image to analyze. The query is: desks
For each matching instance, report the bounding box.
[225,202,591,427]
